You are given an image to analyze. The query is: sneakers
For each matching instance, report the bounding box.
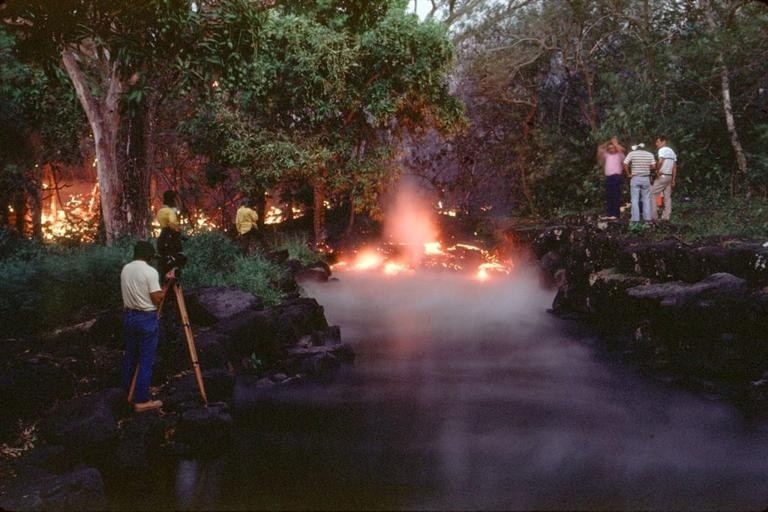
[135,399,161,411]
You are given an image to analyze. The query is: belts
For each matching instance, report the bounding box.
[632,175,650,178]
[123,307,156,313]
[658,173,671,177]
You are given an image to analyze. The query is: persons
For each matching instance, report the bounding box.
[121,241,163,411]
[622,140,656,222]
[597,137,625,221]
[651,136,676,222]
[235,197,269,256]
[157,189,182,255]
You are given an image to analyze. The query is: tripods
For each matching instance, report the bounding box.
[127,268,208,410]
